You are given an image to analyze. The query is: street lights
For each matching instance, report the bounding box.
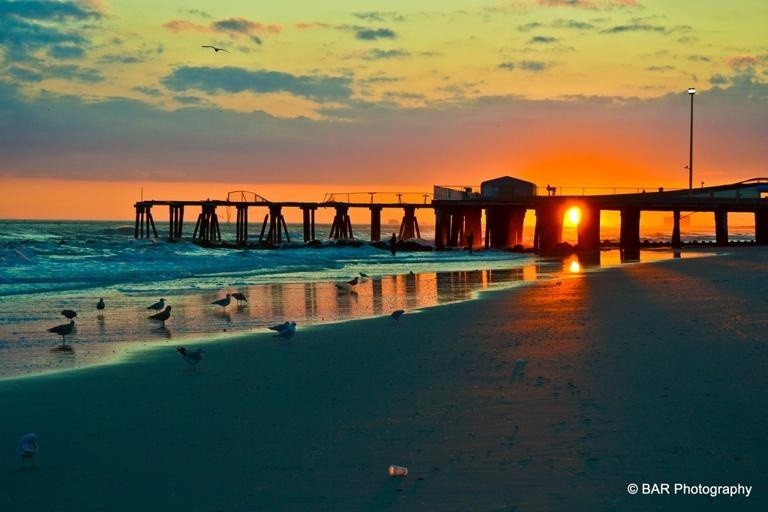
[685,87,697,188]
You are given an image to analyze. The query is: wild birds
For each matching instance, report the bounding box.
[358,271,368,279]
[273,322,298,340]
[19,432,39,468]
[176,347,206,367]
[47,320,75,347]
[390,309,405,323]
[267,321,290,331]
[231,291,247,305]
[347,276,359,285]
[202,45,231,54]
[96,297,105,316]
[211,294,230,309]
[147,297,167,312]
[148,305,172,328]
[60,308,78,322]
[334,284,344,291]
